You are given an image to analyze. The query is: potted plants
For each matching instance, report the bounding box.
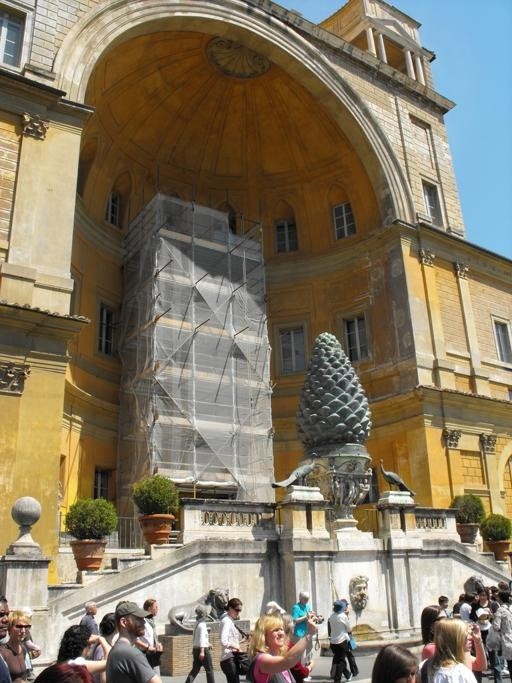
[478,512,512,561]
[449,493,485,544]
[131,474,179,544]
[64,496,118,570]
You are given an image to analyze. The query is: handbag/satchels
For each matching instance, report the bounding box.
[145,648,164,668]
[306,610,318,636]
[230,648,251,675]
[348,632,357,651]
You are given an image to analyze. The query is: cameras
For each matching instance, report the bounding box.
[312,616,324,624]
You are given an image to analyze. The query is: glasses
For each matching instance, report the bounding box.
[12,623,32,630]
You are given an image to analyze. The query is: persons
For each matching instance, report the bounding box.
[372,644,418,682]
[244,613,323,683]
[184,604,216,682]
[0,595,164,683]
[331,475,371,508]
[326,599,359,680]
[219,596,253,682]
[282,615,316,683]
[290,589,317,683]
[414,580,511,683]
[349,575,370,611]
[328,599,354,682]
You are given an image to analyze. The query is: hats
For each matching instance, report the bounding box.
[115,601,154,621]
[194,604,212,622]
[265,601,286,616]
[333,600,347,615]
[227,598,243,612]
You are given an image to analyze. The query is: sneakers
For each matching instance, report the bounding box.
[302,676,313,683]
[347,672,354,681]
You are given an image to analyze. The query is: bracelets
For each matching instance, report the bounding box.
[303,633,311,645]
[308,664,314,672]
